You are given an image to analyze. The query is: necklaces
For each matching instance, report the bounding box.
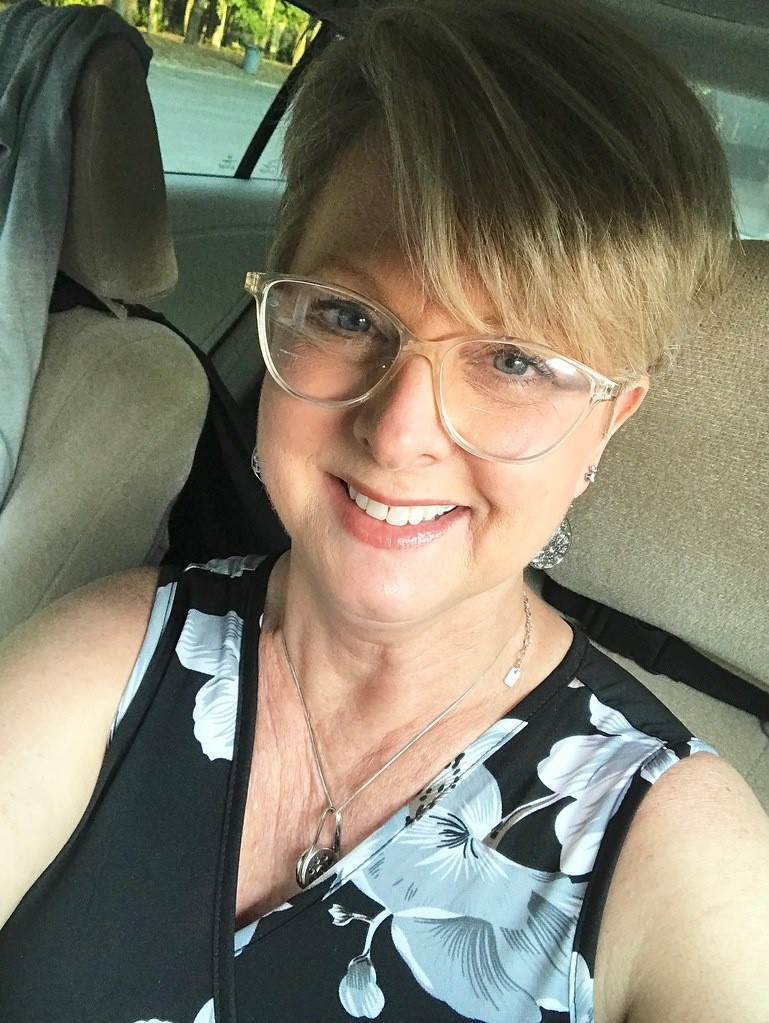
[278,552,533,889]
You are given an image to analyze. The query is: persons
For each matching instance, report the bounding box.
[0,1,768,1023]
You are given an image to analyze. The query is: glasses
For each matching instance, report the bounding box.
[245,272,622,462]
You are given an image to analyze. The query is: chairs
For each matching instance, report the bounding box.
[0,0,213,636]
[525,235,769,815]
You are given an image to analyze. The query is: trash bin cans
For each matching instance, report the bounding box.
[243,45,262,74]
